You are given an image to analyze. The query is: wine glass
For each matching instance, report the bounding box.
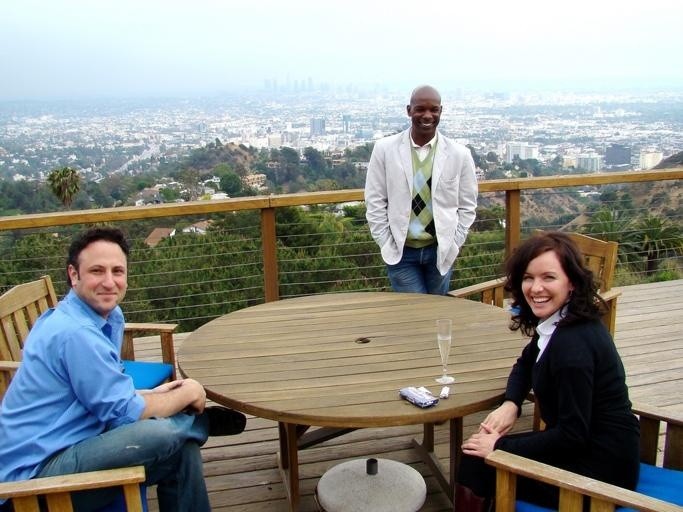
[436,319,458,384]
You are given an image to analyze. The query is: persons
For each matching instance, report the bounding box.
[0,226,248,511]
[454,233,642,511]
[364,85,479,296]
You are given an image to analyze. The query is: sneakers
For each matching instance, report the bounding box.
[203,403,247,439]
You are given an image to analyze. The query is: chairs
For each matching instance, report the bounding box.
[0,274,179,402]
[484,404,683,512]
[1,389,145,512]
[447,229,623,340]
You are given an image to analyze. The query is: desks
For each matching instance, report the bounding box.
[179,291,538,512]
